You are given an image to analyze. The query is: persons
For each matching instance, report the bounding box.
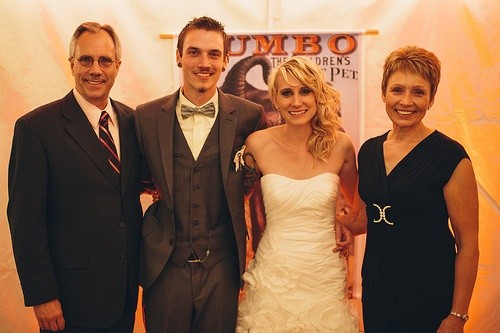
[133,16,352,333]
[151,55,357,333]
[6,22,143,333]
[337,46,480,333]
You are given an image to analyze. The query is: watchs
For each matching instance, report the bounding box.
[450,312,469,321]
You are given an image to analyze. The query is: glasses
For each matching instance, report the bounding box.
[72,55,118,68]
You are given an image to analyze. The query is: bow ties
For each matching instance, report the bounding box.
[181,102,216,120]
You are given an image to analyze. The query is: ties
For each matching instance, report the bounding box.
[98,110,121,175]
